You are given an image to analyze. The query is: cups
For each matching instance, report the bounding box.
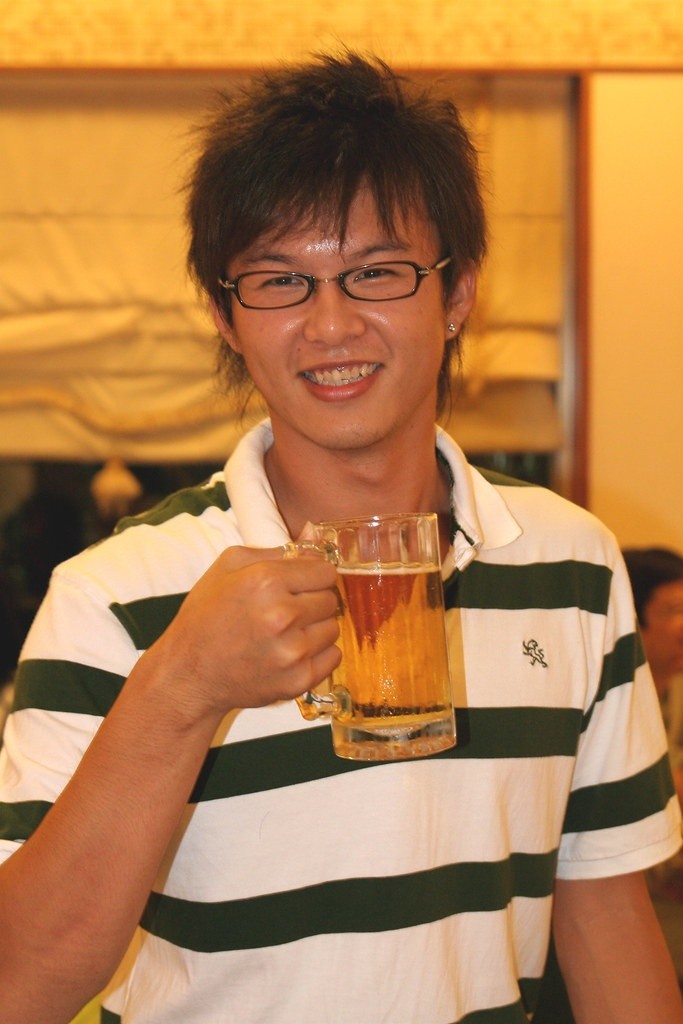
[288,510,461,760]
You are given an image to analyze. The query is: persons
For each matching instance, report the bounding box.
[1,49,683,1024]
[1,465,178,742]
[623,547,683,987]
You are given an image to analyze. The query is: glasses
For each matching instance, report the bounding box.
[218,255,453,310]
